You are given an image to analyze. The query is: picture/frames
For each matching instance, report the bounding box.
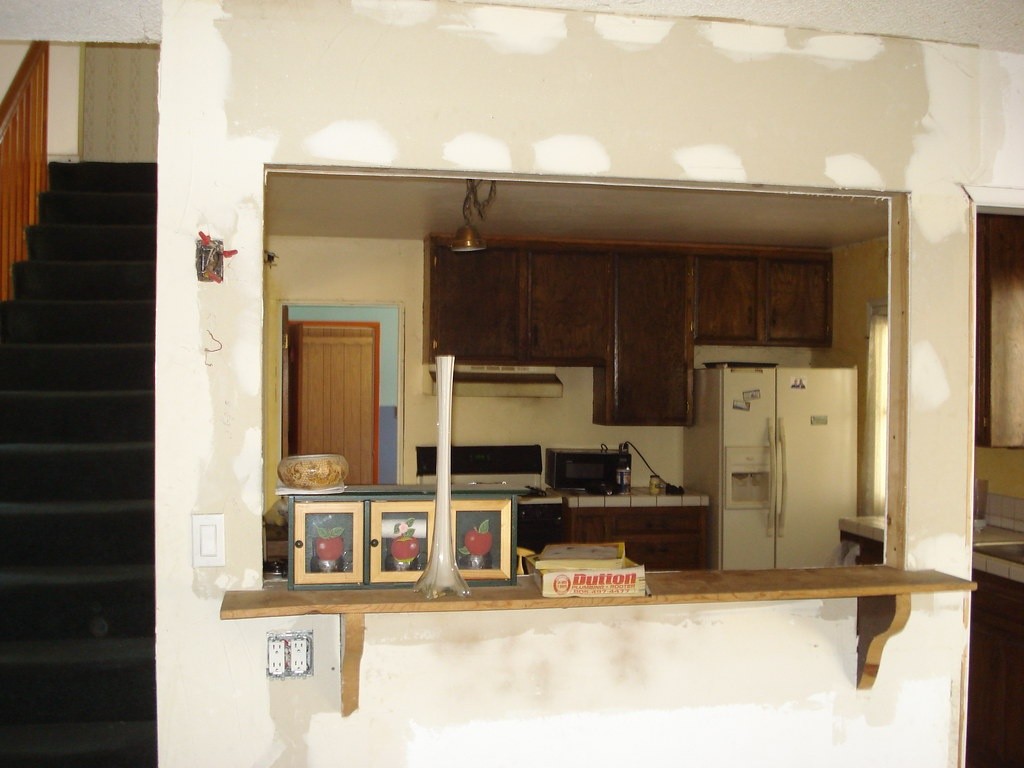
[286,496,517,587]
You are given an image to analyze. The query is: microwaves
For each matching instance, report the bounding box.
[545,447,632,492]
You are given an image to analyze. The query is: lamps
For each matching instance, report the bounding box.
[449,179,496,252]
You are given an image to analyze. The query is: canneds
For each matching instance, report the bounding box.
[616,469,632,494]
[649,475,660,495]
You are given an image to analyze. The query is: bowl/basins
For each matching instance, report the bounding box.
[277,454,349,489]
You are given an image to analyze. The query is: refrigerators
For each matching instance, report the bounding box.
[682,364,858,570]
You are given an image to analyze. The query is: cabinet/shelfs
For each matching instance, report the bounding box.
[428,237,831,425]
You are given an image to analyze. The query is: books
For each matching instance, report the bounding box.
[527,554,646,598]
[534,542,626,570]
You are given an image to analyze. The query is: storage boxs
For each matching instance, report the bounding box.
[528,555,645,597]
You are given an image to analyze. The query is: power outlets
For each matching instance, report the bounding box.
[266,629,315,679]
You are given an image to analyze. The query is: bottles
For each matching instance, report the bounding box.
[649,475,660,495]
